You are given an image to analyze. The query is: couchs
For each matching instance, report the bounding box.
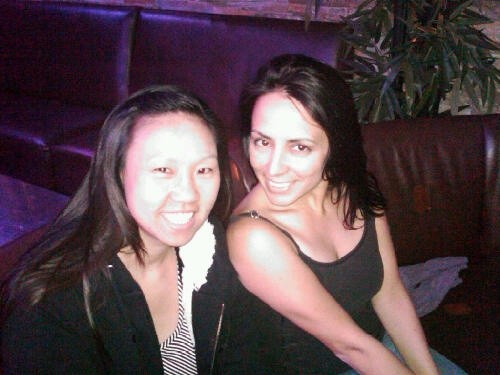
[0,0,355,197]
[228,113,499,375]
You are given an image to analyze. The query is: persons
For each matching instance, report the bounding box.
[0,86,233,375]
[226,54,466,374]
